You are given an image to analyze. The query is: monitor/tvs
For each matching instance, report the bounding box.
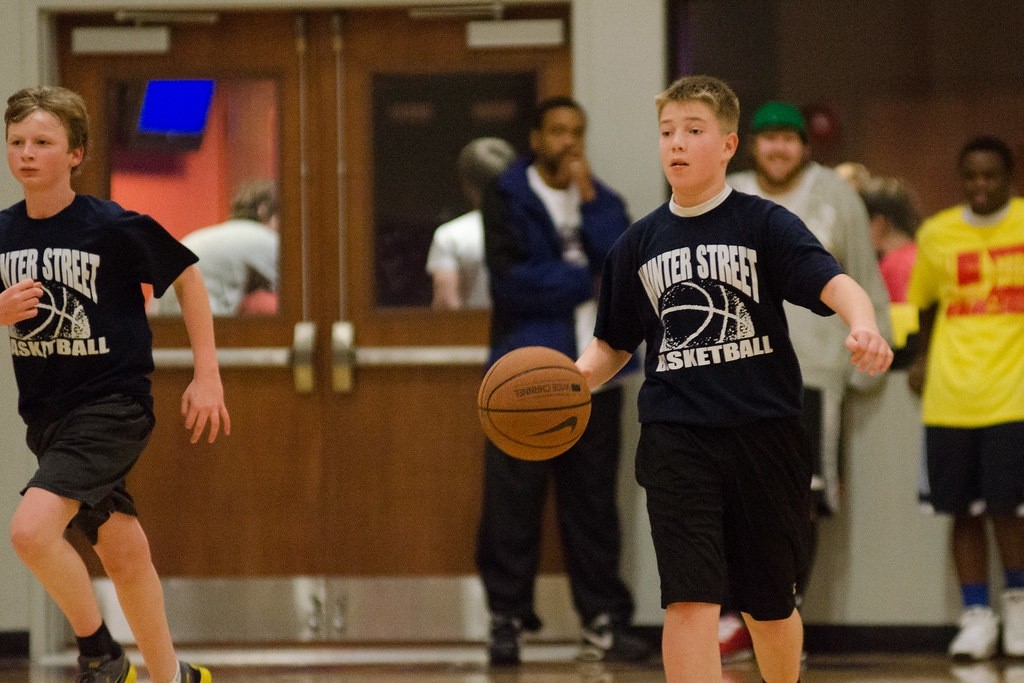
[139,79,215,137]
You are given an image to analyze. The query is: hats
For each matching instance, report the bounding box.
[747,100,806,134]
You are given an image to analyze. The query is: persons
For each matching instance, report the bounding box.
[480,96,650,670]
[426,138,515,310]
[1,86,231,683]
[910,138,1024,658]
[837,164,922,301]
[717,103,891,665]
[150,181,278,317]
[576,76,894,683]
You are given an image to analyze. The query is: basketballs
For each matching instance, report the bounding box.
[477,345,593,463]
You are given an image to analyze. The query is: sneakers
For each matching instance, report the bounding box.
[718,611,753,666]
[486,614,521,665]
[947,607,1001,660]
[579,615,651,660]
[999,587,1024,657]
[75,641,138,683]
[180,660,213,683]
